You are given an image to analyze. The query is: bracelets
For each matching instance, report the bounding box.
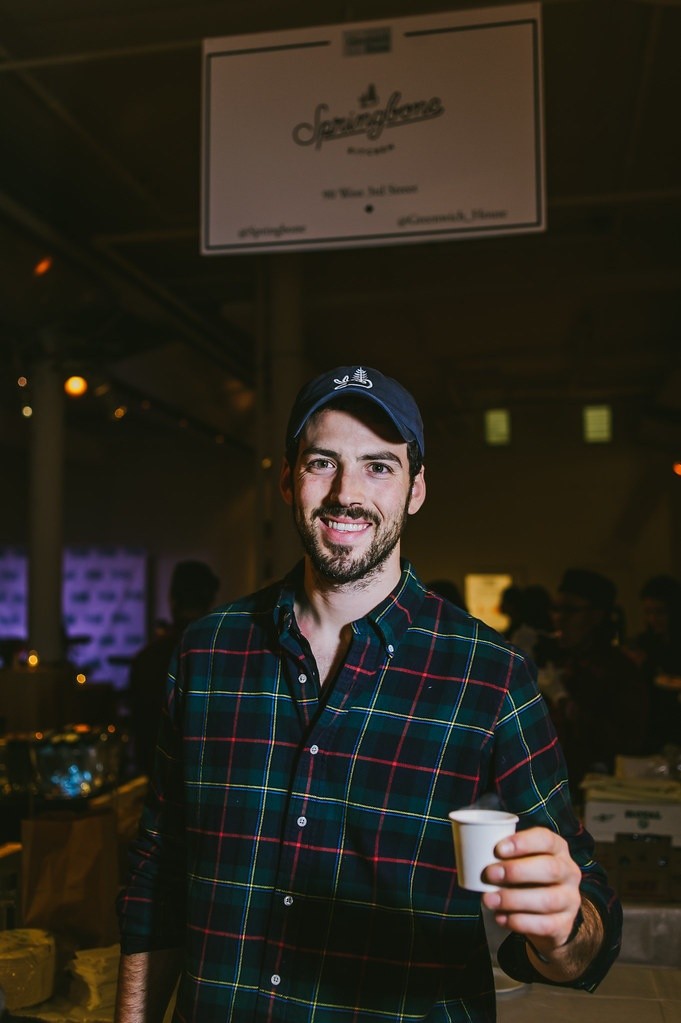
[565,908,584,945]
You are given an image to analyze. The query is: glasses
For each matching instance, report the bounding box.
[551,604,590,615]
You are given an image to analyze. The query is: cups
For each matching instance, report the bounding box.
[448,810,519,893]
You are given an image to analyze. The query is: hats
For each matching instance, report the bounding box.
[286,364,425,457]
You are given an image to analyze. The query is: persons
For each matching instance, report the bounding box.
[127,559,681,805]
[114,363,623,1022]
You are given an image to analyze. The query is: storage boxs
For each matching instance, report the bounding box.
[582,801,681,843]
